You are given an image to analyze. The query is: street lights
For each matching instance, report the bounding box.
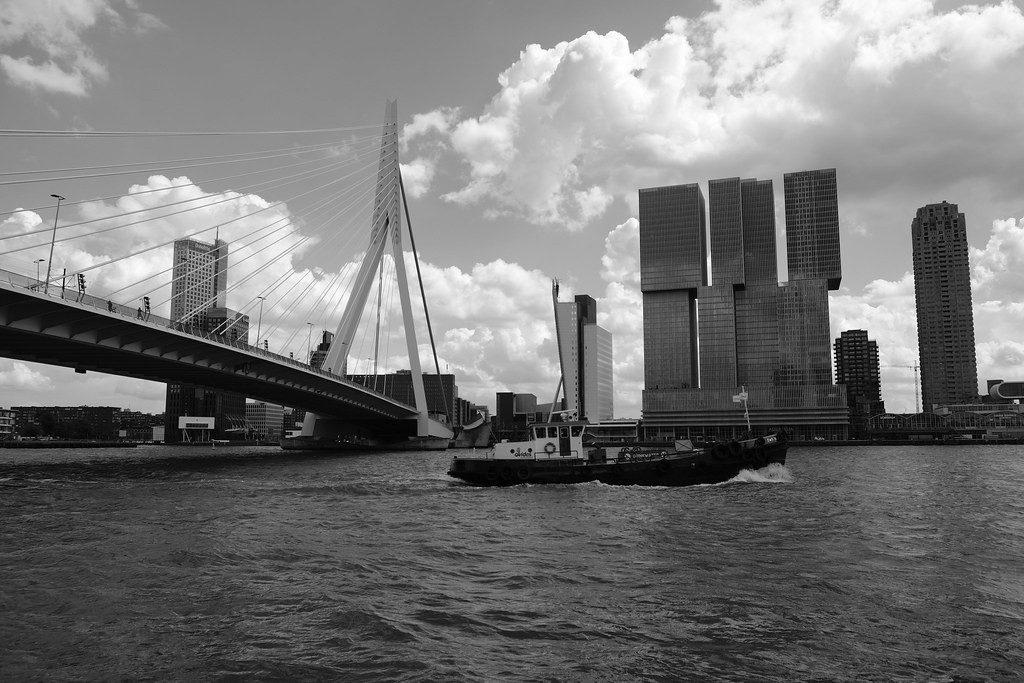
[307,322,315,366]
[43,194,67,296]
[33,258,45,291]
[257,295,266,348]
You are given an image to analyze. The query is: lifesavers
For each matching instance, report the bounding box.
[742,449,755,462]
[728,441,741,456]
[543,442,556,454]
[657,459,673,473]
[711,445,728,460]
[485,464,499,482]
[612,465,625,476]
[518,464,532,479]
[500,463,515,481]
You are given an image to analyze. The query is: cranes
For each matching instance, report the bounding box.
[883,359,924,413]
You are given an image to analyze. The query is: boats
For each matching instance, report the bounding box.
[445,419,791,487]
